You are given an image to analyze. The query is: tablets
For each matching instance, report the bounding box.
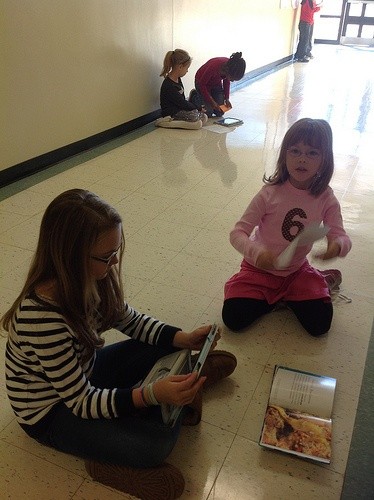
[167,322,218,428]
[139,348,189,390]
[214,117,240,125]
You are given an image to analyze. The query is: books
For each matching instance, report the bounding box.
[258,364,336,465]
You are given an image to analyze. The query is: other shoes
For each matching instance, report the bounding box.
[188,88,197,103]
[306,53,313,59]
[85,457,185,500]
[190,350,238,390]
[155,115,171,127]
[319,269,342,291]
[294,56,310,62]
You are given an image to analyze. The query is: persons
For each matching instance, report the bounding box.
[189,52,246,117]
[294,0,323,63]
[0,189,237,500]
[222,118,351,337]
[155,49,207,129]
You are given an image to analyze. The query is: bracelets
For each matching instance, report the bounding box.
[138,383,158,407]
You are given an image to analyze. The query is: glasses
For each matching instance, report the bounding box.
[87,250,119,268]
[286,146,323,159]
